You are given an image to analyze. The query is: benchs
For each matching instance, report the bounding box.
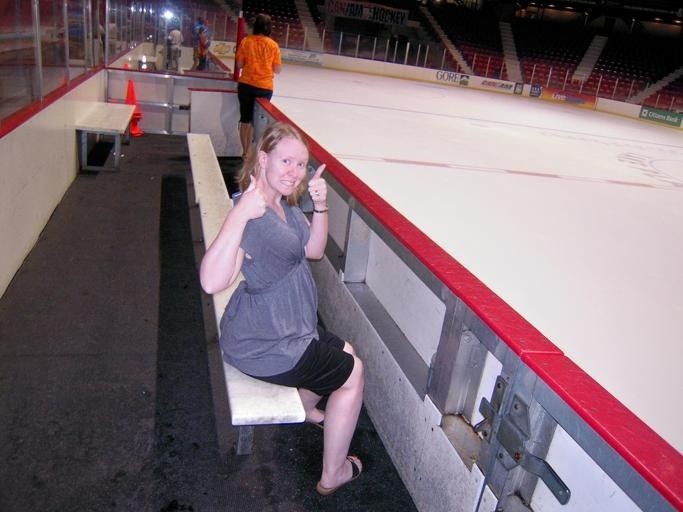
[198,198,307,452]
[184,131,229,206]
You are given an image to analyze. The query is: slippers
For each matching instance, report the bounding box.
[311,408,326,429]
[315,455,363,496]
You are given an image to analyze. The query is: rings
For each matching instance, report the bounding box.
[314,190,320,197]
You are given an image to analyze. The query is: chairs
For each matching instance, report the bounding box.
[172,3,444,69]
[444,2,682,112]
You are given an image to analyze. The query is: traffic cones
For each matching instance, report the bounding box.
[109,78,148,138]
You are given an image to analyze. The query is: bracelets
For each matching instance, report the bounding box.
[313,207,328,213]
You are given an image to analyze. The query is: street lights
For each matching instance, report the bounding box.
[160,8,172,71]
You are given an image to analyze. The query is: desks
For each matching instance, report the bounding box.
[69,100,137,178]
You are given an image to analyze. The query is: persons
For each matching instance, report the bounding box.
[200,121,368,494]
[167,24,185,70]
[191,16,209,69]
[494,66,501,77]
[237,14,282,161]
[197,26,211,69]
[56,13,153,64]
